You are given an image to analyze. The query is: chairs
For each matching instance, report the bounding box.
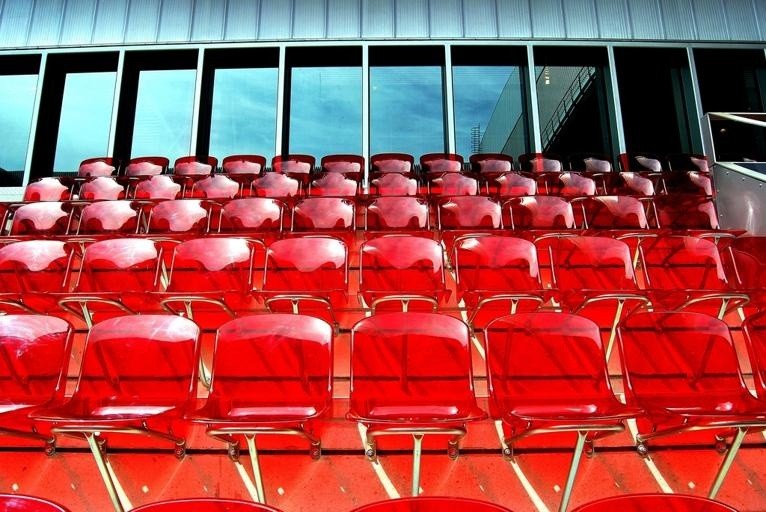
[179,310,336,509]
[348,308,489,504]
[1,311,77,497]
[608,306,764,509]
[2,153,764,397]
[26,312,208,509]
[477,303,642,511]
